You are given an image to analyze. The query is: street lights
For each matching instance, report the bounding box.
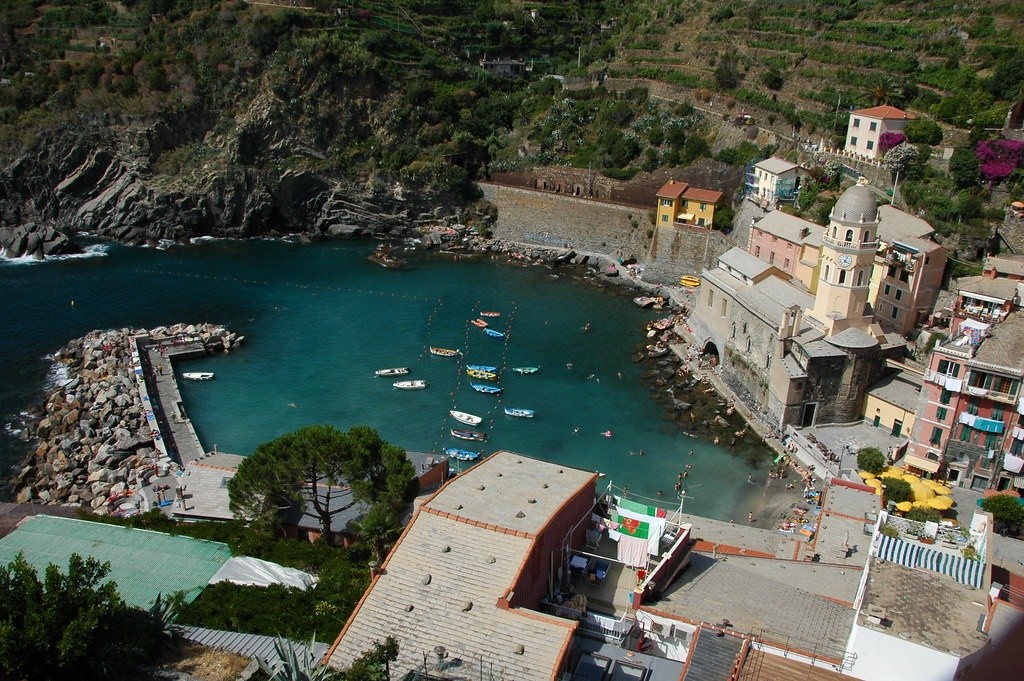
[839,445,850,468]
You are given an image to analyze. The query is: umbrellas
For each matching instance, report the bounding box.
[861,466,952,513]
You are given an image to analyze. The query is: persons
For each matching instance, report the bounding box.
[116,342,176,504]
[566,282,817,530]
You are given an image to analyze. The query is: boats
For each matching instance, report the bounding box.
[429,345,463,356]
[450,410,483,427]
[632,296,676,330]
[375,366,409,377]
[646,347,667,357]
[469,381,505,394]
[467,369,499,380]
[479,311,501,317]
[504,407,535,418]
[451,428,488,442]
[183,371,215,381]
[467,364,501,374]
[483,327,505,337]
[674,398,693,410]
[471,318,489,328]
[512,366,540,375]
[392,380,426,390]
[367,242,410,270]
[442,446,486,460]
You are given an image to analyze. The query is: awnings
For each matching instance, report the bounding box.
[676,211,695,221]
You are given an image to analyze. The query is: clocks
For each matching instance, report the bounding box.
[839,253,852,267]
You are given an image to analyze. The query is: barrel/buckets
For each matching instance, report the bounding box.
[629,593,634,603]
